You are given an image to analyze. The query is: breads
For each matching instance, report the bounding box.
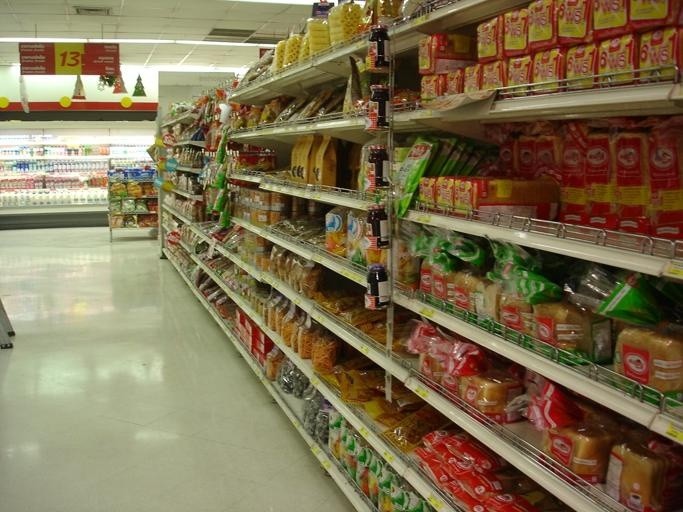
[394,239,683,512]
[477,180,559,223]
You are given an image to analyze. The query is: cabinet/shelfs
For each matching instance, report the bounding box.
[0,102,161,244]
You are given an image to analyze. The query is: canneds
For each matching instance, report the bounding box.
[368,25,390,67]
[368,84,389,126]
[249,291,270,316]
[366,204,389,247]
[366,264,390,307]
[367,145,390,188]
[234,186,318,229]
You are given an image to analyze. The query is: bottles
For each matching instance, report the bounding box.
[0,143,155,206]
